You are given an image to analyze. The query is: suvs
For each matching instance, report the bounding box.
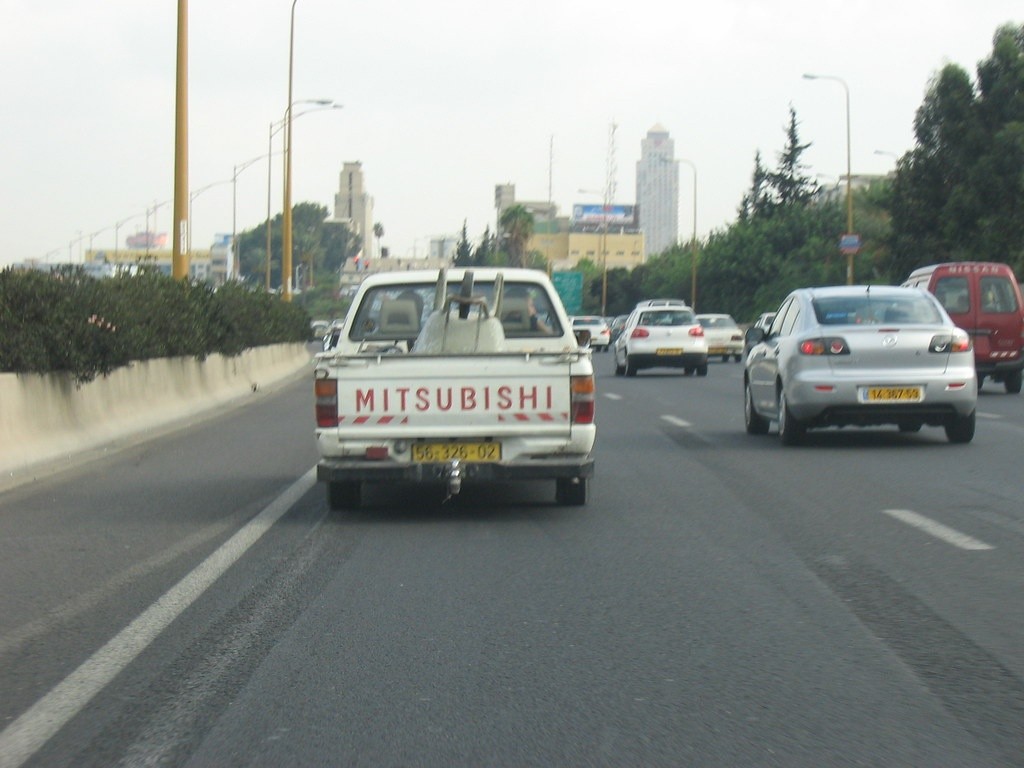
[614,304,709,376]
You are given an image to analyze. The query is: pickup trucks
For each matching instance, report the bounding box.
[310,266,596,508]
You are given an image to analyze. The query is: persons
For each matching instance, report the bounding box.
[506,288,552,334]
[396,290,425,320]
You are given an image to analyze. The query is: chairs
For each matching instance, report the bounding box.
[985,292,997,313]
[643,316,651,325]
[959,291,970,314]
[673,315,685,325]
[501,298,530,332]
[379,298,422,339]
[884,302,911,323]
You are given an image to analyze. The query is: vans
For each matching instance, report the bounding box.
[637,299,686,323]
[900,262,1024,393]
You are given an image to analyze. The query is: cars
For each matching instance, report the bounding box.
[695,314,746,362]
[743,285,979,444]
[570,315,610,351]
[753,312,777,334]
[611,315,629,342]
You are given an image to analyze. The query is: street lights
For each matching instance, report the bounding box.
[266,104,341,294]
[803,73,855,282]
[282,98,332,278]
[188,177,238,262]
[232,151,287,285]
